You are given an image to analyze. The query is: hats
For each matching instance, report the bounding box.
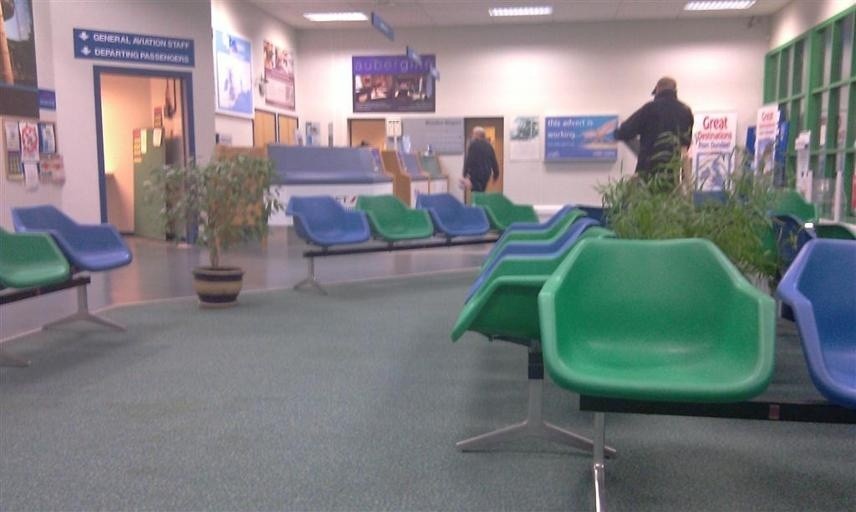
[651,78,676,94]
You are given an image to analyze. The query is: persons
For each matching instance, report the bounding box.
[613,77,694,182]
[462,126,499,193]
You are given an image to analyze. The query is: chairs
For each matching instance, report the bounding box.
[12,204,136,337]
[448,200,620,467]
[0,227,66,297]
[537,235,785,411]
[689,187,856,406]
[285,192,490,300]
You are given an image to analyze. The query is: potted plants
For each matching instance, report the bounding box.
[135,148,287,303]
